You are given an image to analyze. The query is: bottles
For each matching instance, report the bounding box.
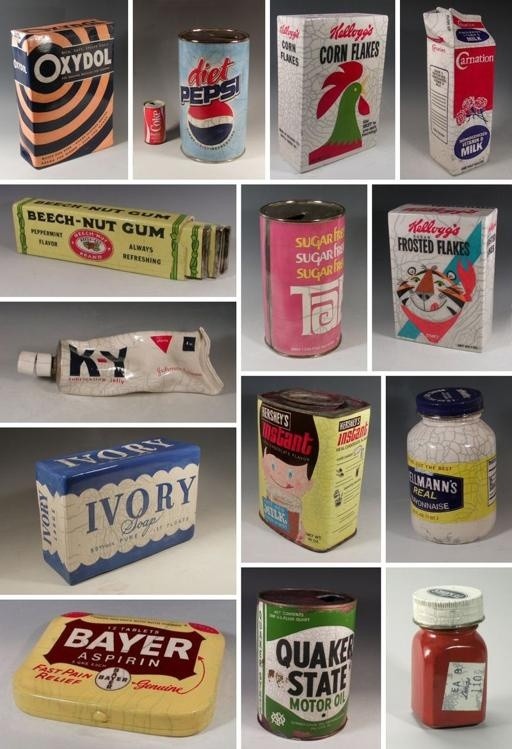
[407,583,489,732]
[404,384,498,547]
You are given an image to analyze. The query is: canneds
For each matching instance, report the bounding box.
[259,199,347,358]
[177,28,250,163]
[255,588,358,742]
[144,100,166,145]
